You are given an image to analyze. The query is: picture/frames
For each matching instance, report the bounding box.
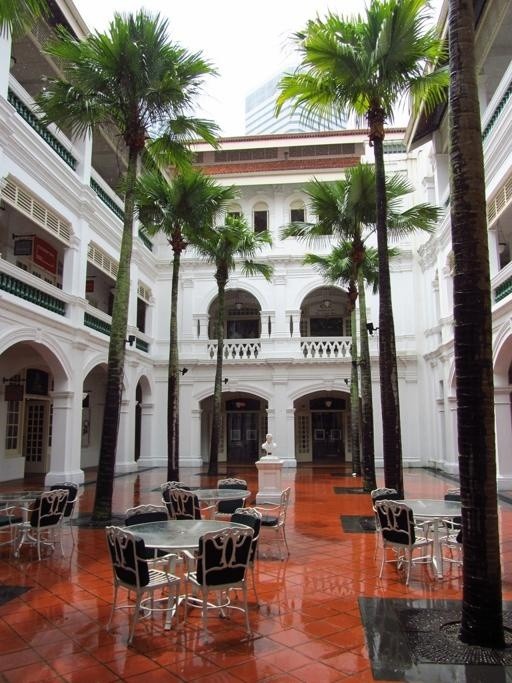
[315,428,325,440]
[247,430,257,440]
[231,430,242,440]
[331,429,341,440]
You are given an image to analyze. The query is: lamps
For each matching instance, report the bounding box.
[124,335,135,346]
[352,359,362,368]
[178,368,188,375]
[221,378,229,385]
[366,322,379,335]
[344,378,350,384]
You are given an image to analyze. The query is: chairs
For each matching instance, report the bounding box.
[1,481,81,564]
[370,486,466,587]
[106,478,290,645]
